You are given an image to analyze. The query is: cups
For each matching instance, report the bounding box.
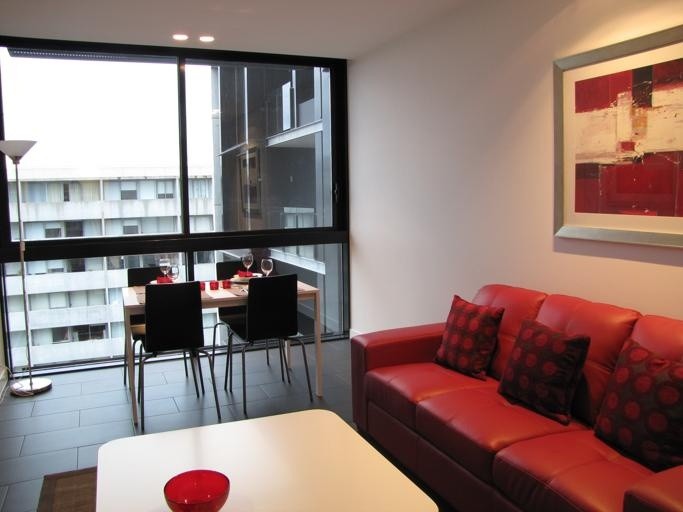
[210,280,219,290]
[200,281,205,290]
[222,280,231,289]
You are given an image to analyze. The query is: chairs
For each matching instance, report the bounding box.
[125,260,314,434]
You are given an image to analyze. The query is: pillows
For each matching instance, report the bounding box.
[429,295,504,385]
[500,317,591,427]
[592,337,682,472]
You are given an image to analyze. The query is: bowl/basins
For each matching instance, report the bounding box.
[164,470,229,511]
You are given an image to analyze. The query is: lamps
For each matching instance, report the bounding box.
[0,140,52,400]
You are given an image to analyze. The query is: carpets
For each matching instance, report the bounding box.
[36,465,97,512]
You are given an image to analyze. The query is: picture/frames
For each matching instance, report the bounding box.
[550,23,683,251]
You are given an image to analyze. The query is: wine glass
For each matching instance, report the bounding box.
[261,258,273,277]
[158,258,170,283]
[166,266,179,283]
[242,253,253,277]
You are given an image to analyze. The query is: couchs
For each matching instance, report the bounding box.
[349,281,681,512]
